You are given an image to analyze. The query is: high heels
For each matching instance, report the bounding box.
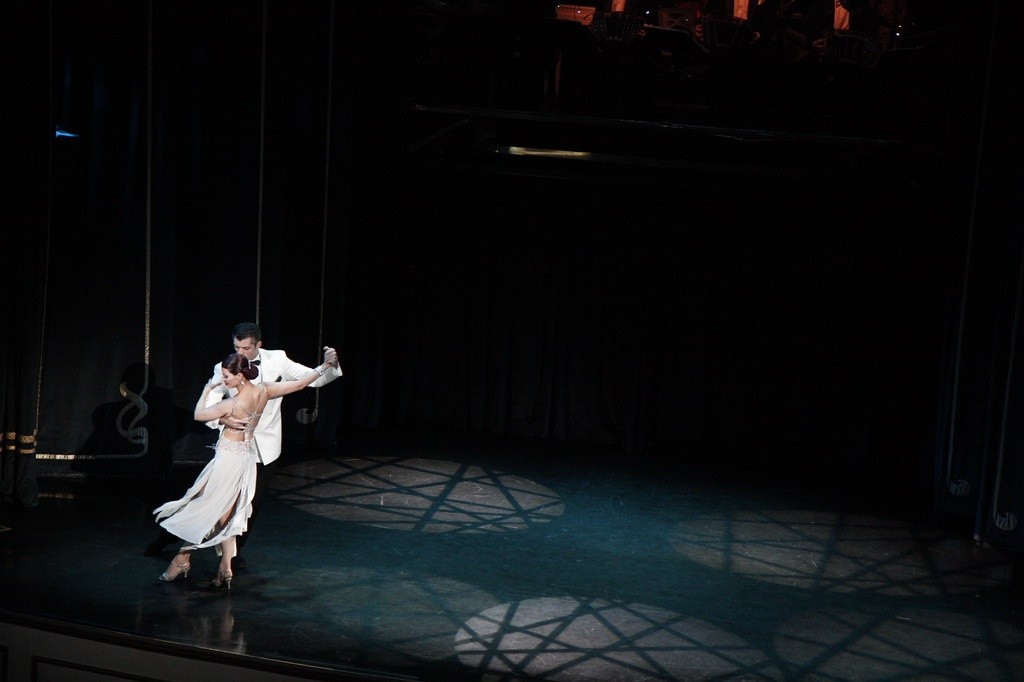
[159,560,191,581]
[212,569,232,590]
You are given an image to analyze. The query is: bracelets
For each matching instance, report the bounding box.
[315,365,325,376]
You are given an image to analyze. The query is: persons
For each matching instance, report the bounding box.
[142,323,343,568]
[152,354,332,591]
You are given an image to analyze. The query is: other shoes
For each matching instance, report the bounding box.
[232,555,247,568]
[148,537,168,555]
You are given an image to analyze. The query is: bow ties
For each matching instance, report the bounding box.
[250,360,261,366]
